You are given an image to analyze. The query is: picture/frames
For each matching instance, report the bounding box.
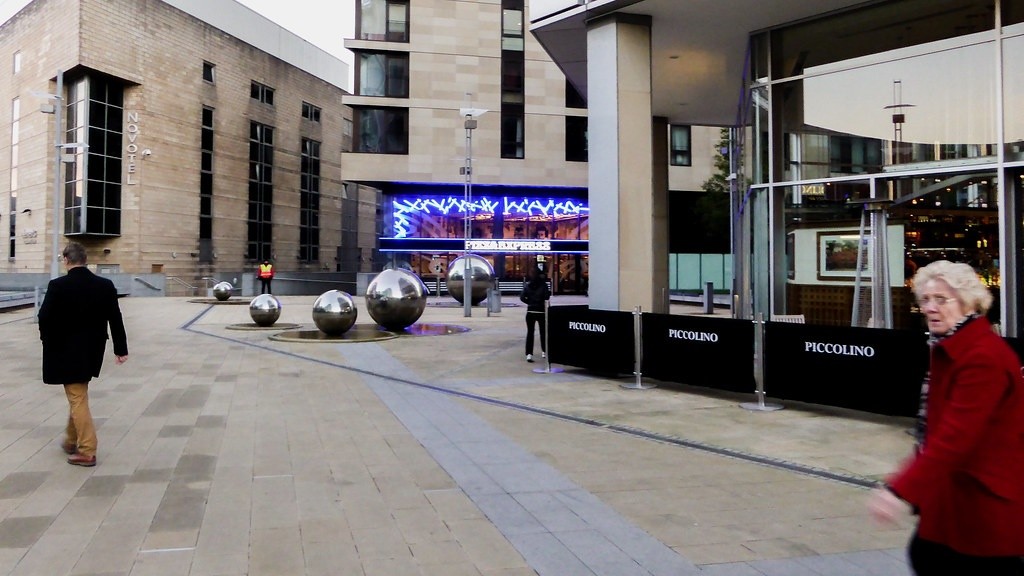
[817,230,872,282]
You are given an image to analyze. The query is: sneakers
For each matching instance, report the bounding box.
[542,351,546,358]
[527,354,535,362]
[68,454,96,467]
[60,441,77,454]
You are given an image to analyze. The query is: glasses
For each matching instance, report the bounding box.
[916,297,961,307]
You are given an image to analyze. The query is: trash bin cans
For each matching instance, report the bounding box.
[489,289,502,312]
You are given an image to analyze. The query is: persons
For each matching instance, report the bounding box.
[518,267,552,361]
[875,259,1022,576]
[35,238,128,467]
[257,258,276,294]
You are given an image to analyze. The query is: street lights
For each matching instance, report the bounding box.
[31,69,66,326]
[458,107,490,318]
